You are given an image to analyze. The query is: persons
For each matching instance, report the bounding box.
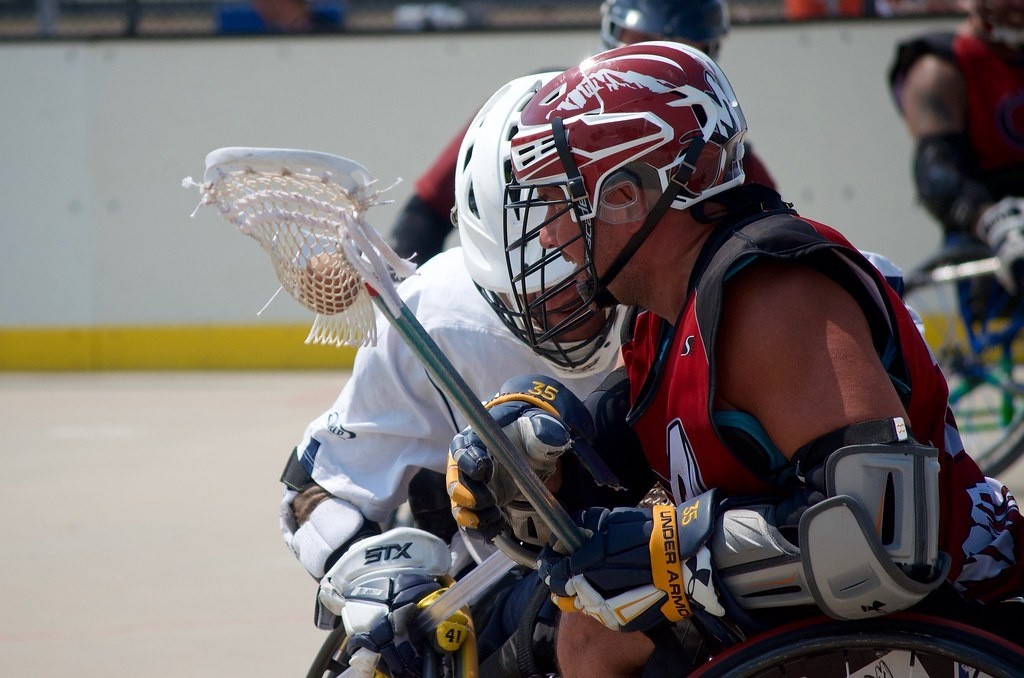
[446,40,1024,678]
[389,0,778,281]
[888,0,1024,299]
[273,72,925,678]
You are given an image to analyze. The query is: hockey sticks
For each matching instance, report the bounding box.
[183,148,587,554]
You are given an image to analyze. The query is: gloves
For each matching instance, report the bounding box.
[978,196,1024,298]
[317,526,479,678]
[538,486,742,634]
[446,374,595,530]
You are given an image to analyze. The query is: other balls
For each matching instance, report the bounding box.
[300,259,354,312]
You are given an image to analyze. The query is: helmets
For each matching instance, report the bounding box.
[600,0,728,63]
[503,40,747,347]
[450,72,630,380]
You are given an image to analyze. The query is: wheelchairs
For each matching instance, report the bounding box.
[308,490,1024,678]
[896,237,1023,485]
